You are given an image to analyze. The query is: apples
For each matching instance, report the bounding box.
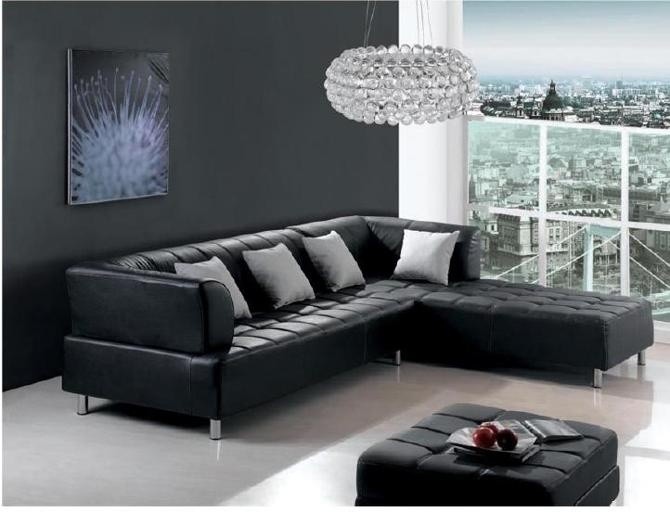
[473,422,518,449]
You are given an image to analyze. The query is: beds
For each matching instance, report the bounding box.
[288,214,654,389]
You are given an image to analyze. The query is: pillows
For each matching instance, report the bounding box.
[240,242,317,310]
[299,229,367,293]
[172,255,253,320]
[390,227,461,288]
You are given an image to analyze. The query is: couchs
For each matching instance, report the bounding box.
[62,228,415,439]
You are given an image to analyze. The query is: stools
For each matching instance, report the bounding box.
[355,403,620,506]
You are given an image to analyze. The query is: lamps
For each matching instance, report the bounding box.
[323,1,481,126]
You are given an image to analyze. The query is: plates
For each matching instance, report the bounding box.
[446,427,537,456]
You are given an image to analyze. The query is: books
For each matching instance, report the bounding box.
[454,441,541,467]
[522,417,584,445]
[479,417,538,444]
